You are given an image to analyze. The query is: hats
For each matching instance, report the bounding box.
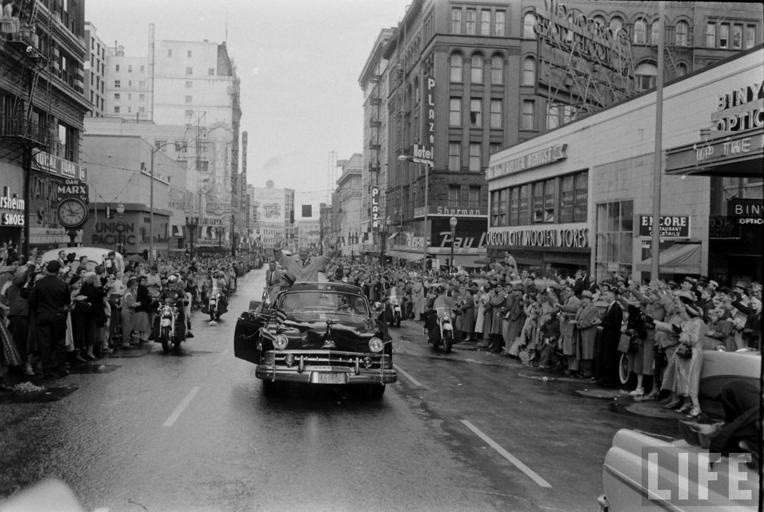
[510,269,762,340]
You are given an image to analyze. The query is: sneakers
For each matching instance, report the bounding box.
[75,331,149,362]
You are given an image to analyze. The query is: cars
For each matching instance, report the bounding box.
[234,282,396,400]
[40,247,125,285]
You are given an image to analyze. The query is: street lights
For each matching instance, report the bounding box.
[448,217,458,267]
[149,142,182,273]
[115,202,127,253]
[397,154,430,276]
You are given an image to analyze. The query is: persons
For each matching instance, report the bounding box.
[0,216,763,418]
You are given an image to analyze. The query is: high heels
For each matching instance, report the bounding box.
[631,386,703,420]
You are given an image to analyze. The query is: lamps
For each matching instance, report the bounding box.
[115,202,126,218]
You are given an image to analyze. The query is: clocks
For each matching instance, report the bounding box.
[57,198,88,228]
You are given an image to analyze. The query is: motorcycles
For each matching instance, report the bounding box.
[202,287,228,321]
[422,297,462,354]
[383,287,408,328]
[149,296,189,352]
